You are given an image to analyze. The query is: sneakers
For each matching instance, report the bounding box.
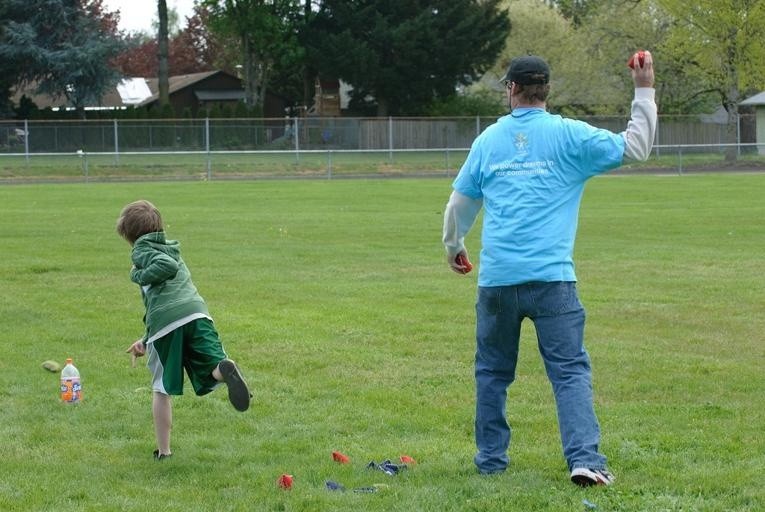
[570,467,616,488]
[218,358,250,412]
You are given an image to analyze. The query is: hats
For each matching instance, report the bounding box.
[499,55,551,85]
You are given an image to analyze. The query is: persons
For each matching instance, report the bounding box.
[115,200,252,461]
[442,50,658,487]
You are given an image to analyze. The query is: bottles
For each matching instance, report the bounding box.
[59,358,82,405]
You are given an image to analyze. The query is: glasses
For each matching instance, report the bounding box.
[505,80,515,89]
